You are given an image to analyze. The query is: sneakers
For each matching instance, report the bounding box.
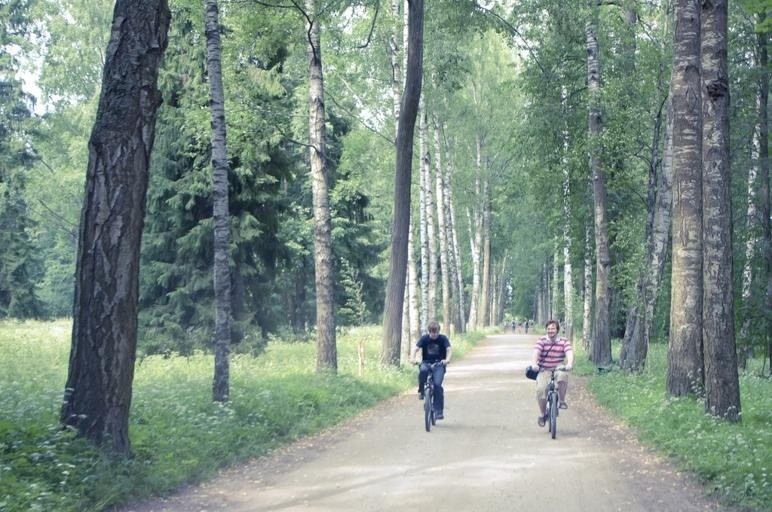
[435,410,443,419]
[559,402,567,409]
[418,392,424,399]
[538,415,545,427]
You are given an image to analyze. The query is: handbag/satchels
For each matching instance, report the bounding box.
[525,366,538,380]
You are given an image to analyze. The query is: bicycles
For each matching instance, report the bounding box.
[410,361,449,432]
[531,366,573,439]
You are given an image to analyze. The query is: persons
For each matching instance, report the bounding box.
[530,319,575,427]
[503,318,535,336]
[409,322,451,419]
